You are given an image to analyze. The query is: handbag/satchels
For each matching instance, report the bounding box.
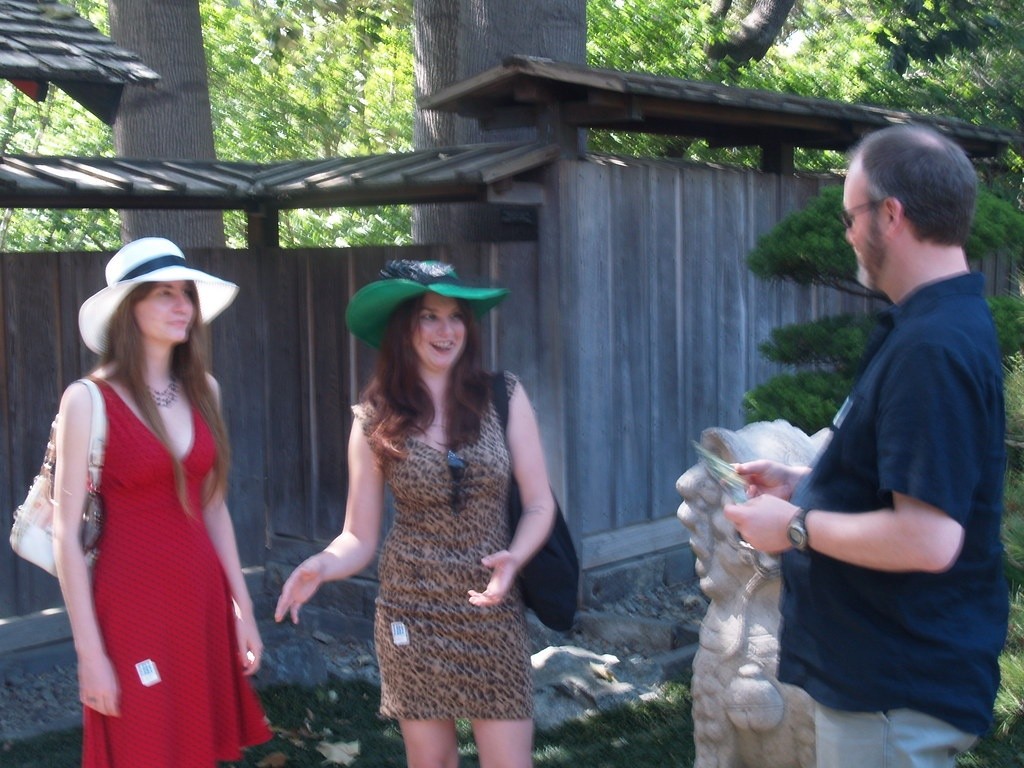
[493,368,581,632]
[9,380,102,582]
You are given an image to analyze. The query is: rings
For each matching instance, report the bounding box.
[87,695,97,703]
[50,236,272,768]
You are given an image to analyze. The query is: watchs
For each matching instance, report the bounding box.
[786,508,812,554]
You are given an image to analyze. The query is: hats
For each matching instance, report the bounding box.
[78,236,239,357]
[347,263,513,352]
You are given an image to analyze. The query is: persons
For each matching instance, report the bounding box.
[275,259,559,768]
[723,126,1011,768]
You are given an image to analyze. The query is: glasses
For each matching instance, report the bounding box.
[445,450,469,514]
[837,201,873,230]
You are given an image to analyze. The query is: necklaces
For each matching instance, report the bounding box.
[145,371,177,408]
